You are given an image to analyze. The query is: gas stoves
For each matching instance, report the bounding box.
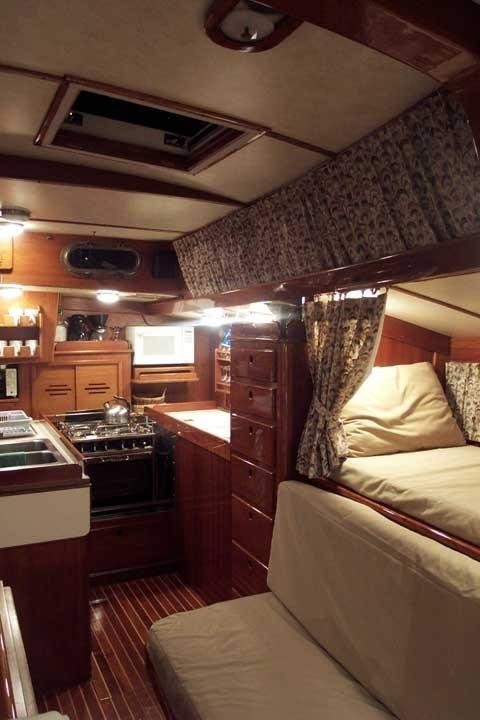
[58,419,155,452]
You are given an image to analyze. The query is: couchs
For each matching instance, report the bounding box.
[144,477,479,720]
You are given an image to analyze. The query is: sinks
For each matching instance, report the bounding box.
[1,438,61,455]
[1,451,75,471]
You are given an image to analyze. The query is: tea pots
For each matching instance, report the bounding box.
[103,395,131,423]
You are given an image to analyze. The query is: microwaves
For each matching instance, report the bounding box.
[0,363,19,399]
[126,326,194,364]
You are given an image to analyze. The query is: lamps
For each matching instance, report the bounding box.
[0,208,32,239]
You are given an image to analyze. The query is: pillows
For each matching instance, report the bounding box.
[340,361,465,456]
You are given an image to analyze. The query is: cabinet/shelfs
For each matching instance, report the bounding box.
[214,348,231,393]
[29,340,133,419]
[1,305,44,361]
[230,317,306,594]
[173,437,231,605]
[87,510,174,572]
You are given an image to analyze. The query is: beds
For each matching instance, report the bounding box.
[313,313,480,563]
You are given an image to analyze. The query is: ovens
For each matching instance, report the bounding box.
[85,456,176,516]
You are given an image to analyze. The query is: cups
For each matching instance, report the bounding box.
[9,340,23,356]
[25,340,39,355]
[0,340,8,355]
